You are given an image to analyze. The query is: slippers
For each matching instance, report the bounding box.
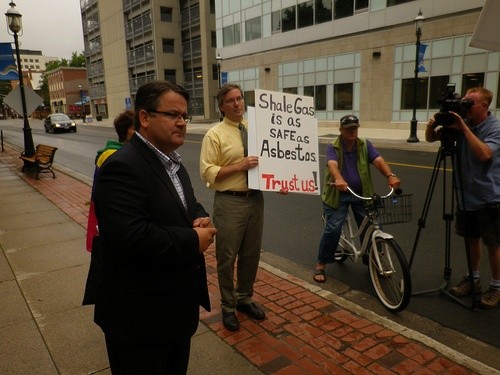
[313,269,327,283]
[368,262,390,280]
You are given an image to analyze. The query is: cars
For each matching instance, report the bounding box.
[44,113,77,134]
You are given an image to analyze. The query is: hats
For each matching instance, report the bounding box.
[340,114,359,128]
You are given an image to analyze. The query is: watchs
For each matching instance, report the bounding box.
[387,173,396,178]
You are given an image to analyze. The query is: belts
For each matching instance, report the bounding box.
[218,190,262,198]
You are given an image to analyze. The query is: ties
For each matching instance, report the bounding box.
[238,122,248,187]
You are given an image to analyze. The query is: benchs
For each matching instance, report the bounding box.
[18,144,58,180]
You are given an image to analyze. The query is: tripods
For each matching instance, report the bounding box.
[406,130,477,310]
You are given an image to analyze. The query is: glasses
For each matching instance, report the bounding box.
[145,108,192,124]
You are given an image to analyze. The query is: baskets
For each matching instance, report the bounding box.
[363,193,415,226]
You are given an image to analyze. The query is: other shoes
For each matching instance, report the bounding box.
[236,303,266,320]
[222,308,240,331]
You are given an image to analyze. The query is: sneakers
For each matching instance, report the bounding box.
[449,276,482,298]
[479,286,500,309]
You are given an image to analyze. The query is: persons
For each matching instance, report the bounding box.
[426,87,500,307]
[200,83,265,330]
[86,111,143,252]
[313,114,400,282]
[81,80,217,375]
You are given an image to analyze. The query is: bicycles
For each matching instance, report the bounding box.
[321,181,411,313]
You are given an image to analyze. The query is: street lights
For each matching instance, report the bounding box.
[215,52,222,123]
[4,0,35,156]
[407,7,425,142]
[78,84,86,122]
